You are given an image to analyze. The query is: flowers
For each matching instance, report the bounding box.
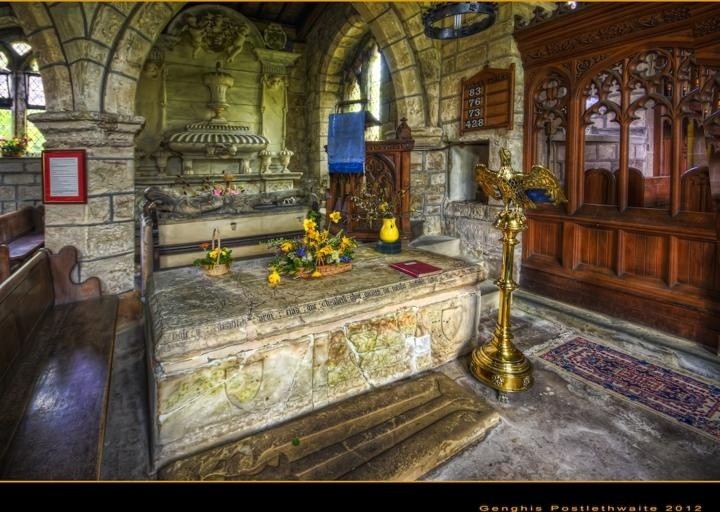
[347,177,427,224]
[0,134,33,156]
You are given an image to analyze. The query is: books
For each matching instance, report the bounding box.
[389,260,443,278]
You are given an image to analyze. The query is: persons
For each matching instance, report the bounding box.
[181,14,215,61]
[225,25,255,65]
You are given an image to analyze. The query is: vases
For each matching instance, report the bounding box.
[378,217,400,243]
[1,151,22,158]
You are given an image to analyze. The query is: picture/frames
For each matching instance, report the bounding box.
[41,150,87,205]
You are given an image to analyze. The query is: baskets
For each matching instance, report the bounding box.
[199,227,230,276]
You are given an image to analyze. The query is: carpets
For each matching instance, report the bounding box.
[526,329,703,436]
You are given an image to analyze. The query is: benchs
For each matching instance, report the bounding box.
[1,199,120,480]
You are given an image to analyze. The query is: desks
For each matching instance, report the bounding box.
[144,243,488,468]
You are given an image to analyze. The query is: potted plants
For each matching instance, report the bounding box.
[267,204,360,286]
